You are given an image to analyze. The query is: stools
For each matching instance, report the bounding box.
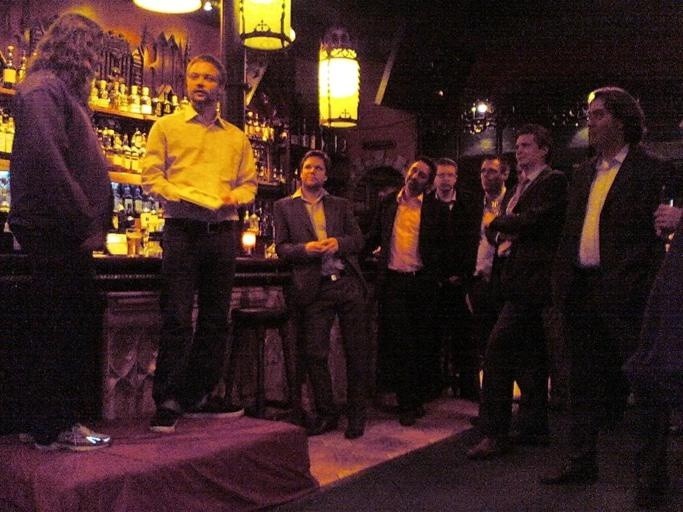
[225,304,304,425]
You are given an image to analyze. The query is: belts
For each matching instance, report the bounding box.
[385,271,430,279]
[162,216,238,237]
[318,270,353,285]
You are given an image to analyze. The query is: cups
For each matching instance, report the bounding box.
[657,199,677,235]
[486,198,501,215]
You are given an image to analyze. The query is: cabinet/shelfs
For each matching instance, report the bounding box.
[276,108,352,200]
[0,85,282,195]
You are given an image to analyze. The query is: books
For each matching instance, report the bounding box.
[171,187,224,212]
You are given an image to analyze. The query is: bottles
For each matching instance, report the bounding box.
[1,44,30,152]
[243,110,333,237]
[87,77,189,257]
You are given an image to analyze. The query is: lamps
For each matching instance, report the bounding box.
[238,0,292,52]
[311,18,361,132]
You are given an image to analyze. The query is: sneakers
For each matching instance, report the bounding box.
[344,406,369,440]
[540,457,600,486]
[149,395,183,435]
[502,424,552,448]
[466,432,515,461]
[181,393,245,420]
[399,405,416,427]
[299,412,341,438]
[33,420,115,453]
[633,461,670,508]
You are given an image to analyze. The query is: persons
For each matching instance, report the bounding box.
[272,150,369,440]
[366,157,451,426]
[7,12,113,455]
[141,52,258,436]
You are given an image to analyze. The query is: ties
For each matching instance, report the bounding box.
[497,176,531,259]
